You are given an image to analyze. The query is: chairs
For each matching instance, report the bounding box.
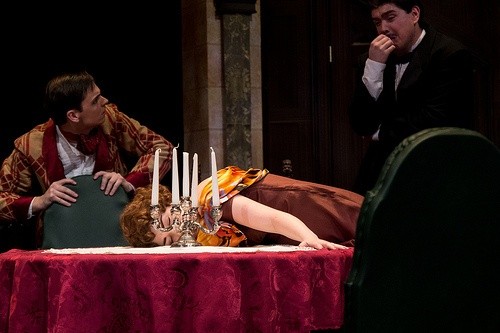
[43,175,131,249]
[343,126,500,333]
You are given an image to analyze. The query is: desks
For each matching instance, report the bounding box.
[1,246,354,332]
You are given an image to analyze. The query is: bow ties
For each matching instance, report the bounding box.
[392,51,413,64]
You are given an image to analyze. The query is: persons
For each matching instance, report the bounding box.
[352,0,473,195]
[0,72,174,249]
[120,165,365,250]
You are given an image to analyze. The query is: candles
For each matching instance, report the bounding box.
[191,153,198,208]
[182,152,190,199]
[151,149,161,206]
[172,143,180,203]
[210,147,219,206]
